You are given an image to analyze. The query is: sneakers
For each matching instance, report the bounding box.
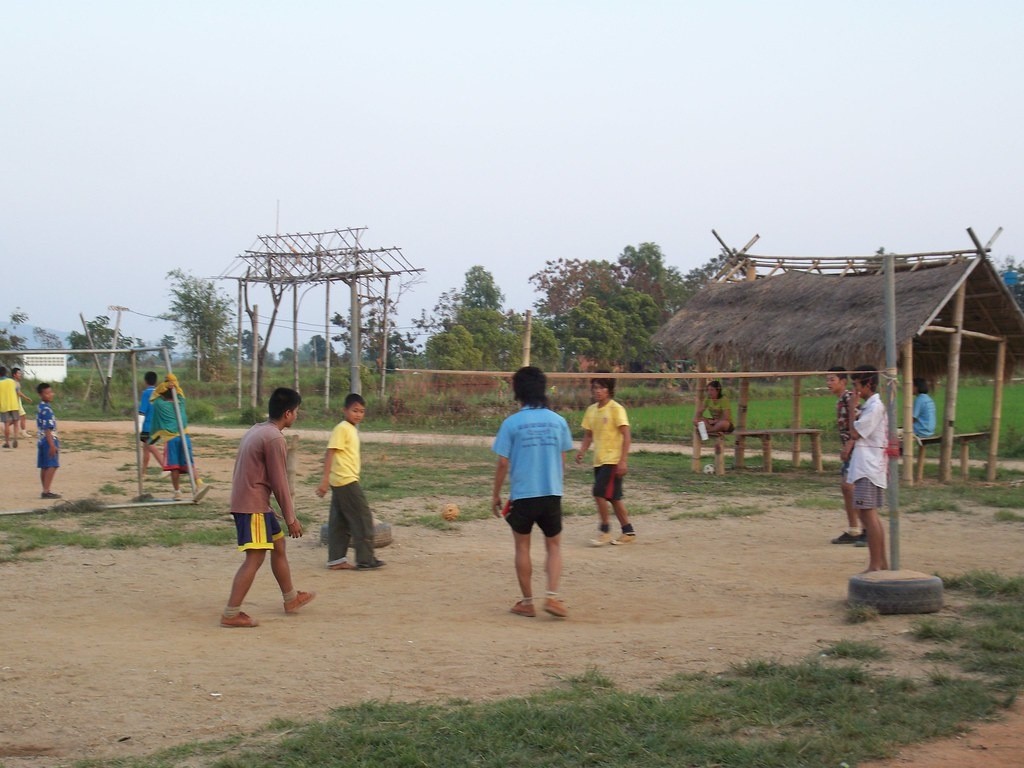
[283,591,316,613]
[510,601,536,617]
[543,598,568,617]
[831,529,869,547]
[219,611,259,628]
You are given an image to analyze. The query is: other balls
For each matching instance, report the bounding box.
[441,503,459,521]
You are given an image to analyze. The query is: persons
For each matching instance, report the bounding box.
[315,393,387,571]
[845,367,889,572]
[692,381,735,438]
[574,370,638,546]
[897,376,936,456]
[491,366,573,617]
[220,387,316,628]
[36,383,62,499]
[1003,264,1020,293]
[147,375,211,502]
[132,371,171,480]
[0,367,33,449]
[826,367,869,546]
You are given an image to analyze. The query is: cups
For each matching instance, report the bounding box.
[697,421,709,440]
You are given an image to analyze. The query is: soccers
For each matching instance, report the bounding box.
[704,463,716,475]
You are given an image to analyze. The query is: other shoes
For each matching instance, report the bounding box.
[172,490,181,500]
[610,534,636,545]
[356,560,386,571]
[2,443,9,448]
[192,483,209,502]
[159,470,172,479]
[21,430,32,438]
[41,492,62,498]
[589,531,610,546]
[13,440,17,447]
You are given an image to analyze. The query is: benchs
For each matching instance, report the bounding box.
[692,428,823,476]
[897,431,992,485]
